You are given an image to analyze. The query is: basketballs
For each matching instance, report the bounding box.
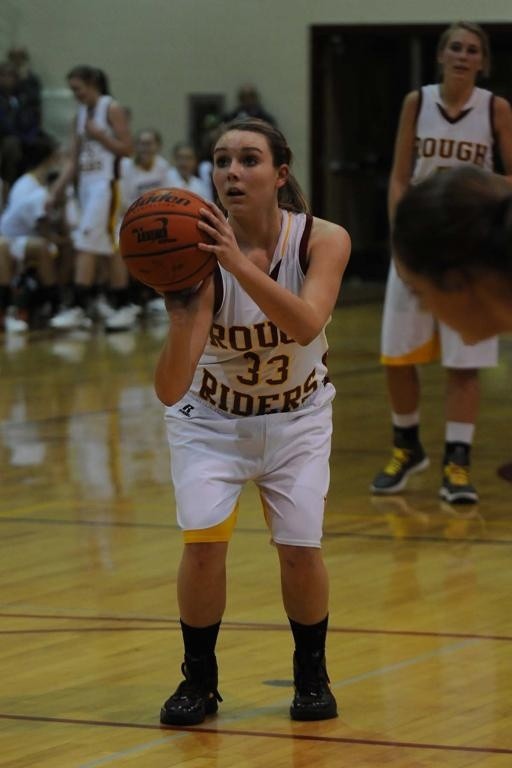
[120,187,217,292]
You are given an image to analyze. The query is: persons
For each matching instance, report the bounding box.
[369,20,511,504]
[154,116,354,725]
[0,45,276,333]
[390,167,512,345]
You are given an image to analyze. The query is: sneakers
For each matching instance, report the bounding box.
[439,460,479,503]
[160,656,223,724]
[372,445,430,493]
[290,650,337,720]
[50,295,167,330]
